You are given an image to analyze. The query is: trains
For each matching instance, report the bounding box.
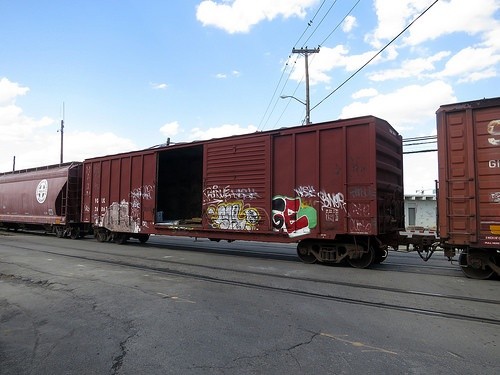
[0,98,500,280]
